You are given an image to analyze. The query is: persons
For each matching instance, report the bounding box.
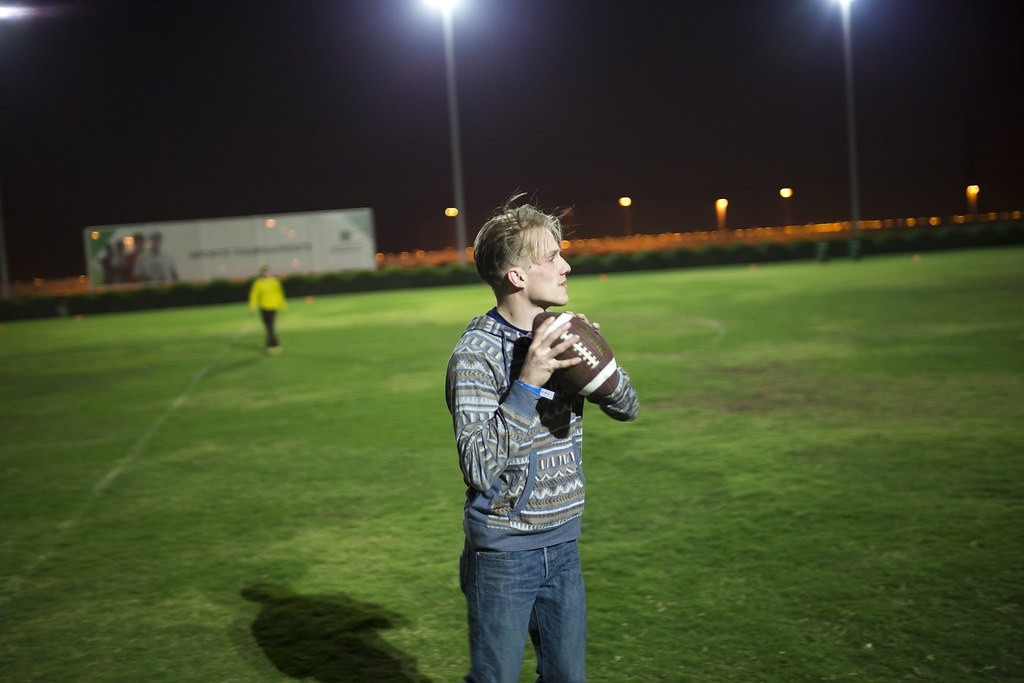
[445,204,638,683]
[96,230,177,286]
[247,265,286,351]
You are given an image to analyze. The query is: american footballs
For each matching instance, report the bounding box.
[533,311,620,400]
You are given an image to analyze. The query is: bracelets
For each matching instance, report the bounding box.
[516,380,554,400]
